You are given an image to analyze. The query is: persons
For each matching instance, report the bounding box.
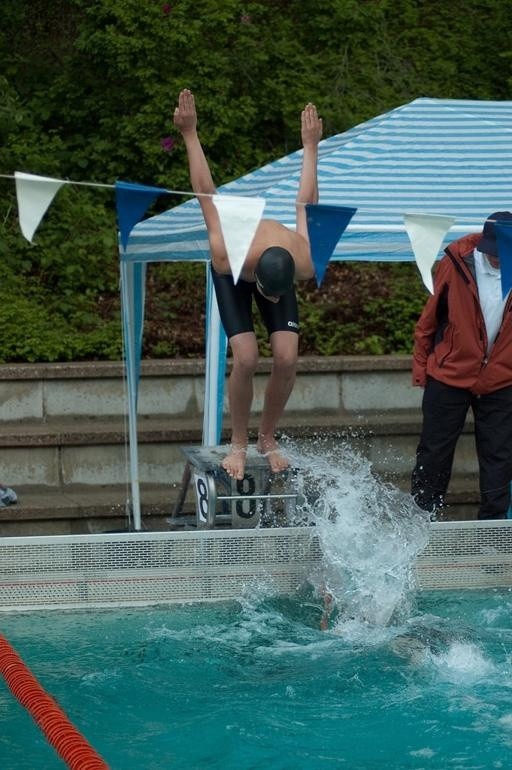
[168,85,323,482]
[411,210,512,573]
[318,581,470,669]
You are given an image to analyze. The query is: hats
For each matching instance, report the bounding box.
[254,246,295,297]
[477,212,512,256]
